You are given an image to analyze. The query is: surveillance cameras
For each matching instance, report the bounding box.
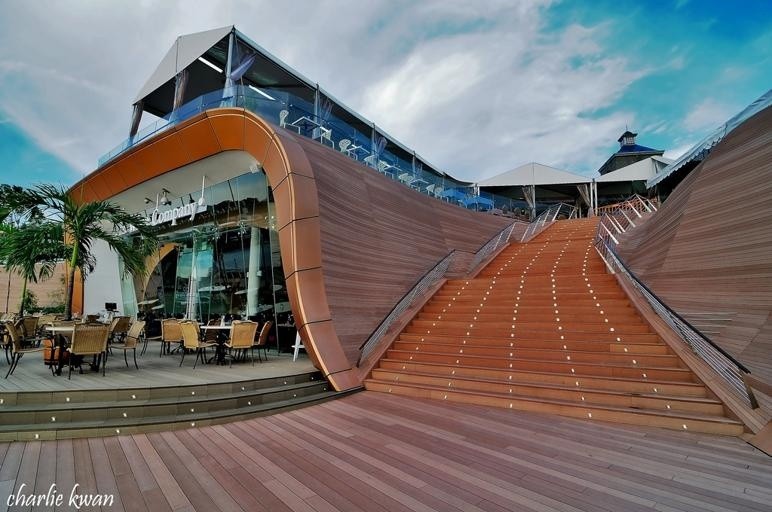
[161,197,168,205]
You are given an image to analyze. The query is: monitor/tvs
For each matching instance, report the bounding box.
[106,303,116,311]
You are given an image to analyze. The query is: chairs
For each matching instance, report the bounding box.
[1,309,307,377]
[278,109,533,223]
